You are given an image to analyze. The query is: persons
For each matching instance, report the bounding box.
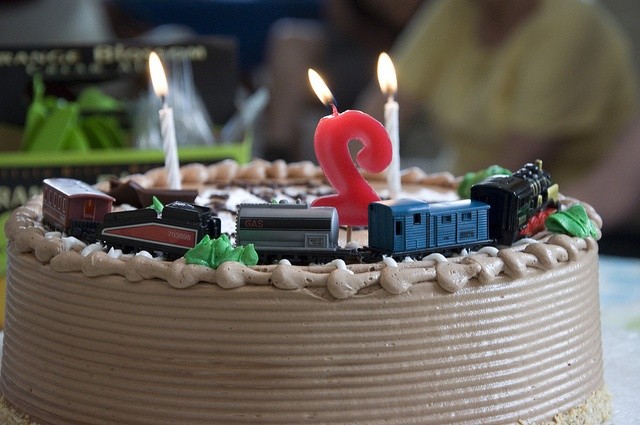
[218,19,332,164]
[352,0,639,195]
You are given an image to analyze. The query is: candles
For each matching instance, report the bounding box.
[145,50,191,191]
[375,50,403,192]
[306,65,393,228]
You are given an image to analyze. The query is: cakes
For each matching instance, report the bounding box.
[1,158,603,425]
